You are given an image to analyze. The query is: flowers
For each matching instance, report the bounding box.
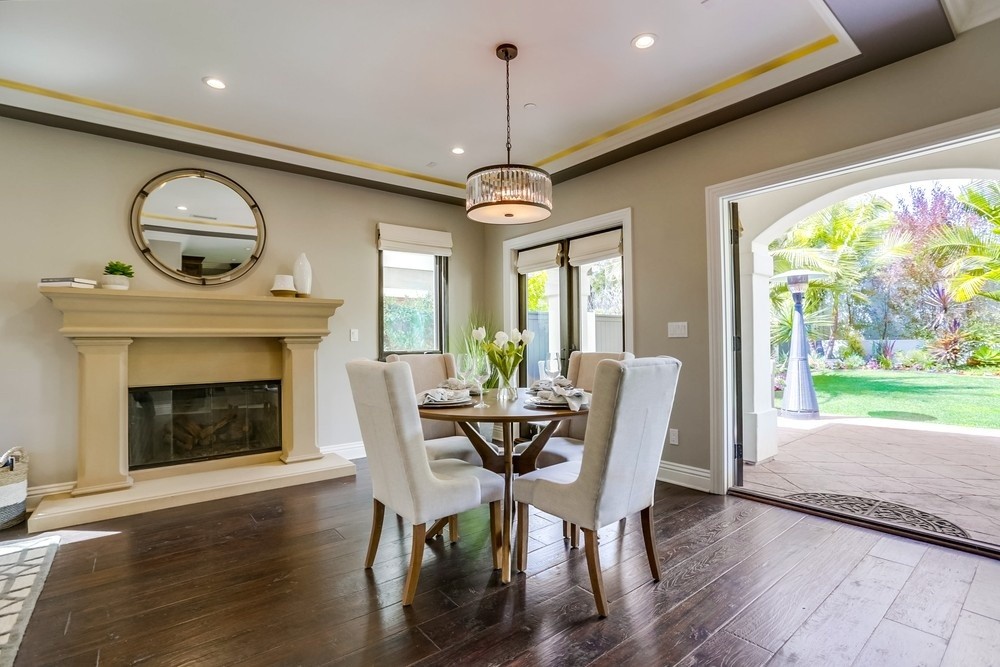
[472,325,535,403]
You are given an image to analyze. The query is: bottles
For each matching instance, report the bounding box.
[294,252,313,298]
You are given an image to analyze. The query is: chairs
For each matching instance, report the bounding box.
[345,351,682,617]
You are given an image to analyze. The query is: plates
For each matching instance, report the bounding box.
[422,387,489,407]
[524,390,569,408]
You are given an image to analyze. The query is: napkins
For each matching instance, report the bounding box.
[532,386,592,411]
[421,388,469,404]
[439,376,482,392]
[530,375,572,389]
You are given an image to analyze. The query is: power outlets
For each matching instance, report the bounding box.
[670,428,679,445]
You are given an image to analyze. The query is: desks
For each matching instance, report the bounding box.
[417,387,599,542]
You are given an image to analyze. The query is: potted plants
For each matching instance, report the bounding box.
[102,260,135,290]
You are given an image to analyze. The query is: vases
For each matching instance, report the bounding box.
[270,252,313,297]
[495,366,519,402]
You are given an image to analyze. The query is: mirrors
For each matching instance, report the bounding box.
[130,168,266,285]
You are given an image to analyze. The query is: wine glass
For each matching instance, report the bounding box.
[456,354,472,387]
[471,355,491,407]
[544,352,561,388]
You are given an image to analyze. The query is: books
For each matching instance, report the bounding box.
[38,277,97,288]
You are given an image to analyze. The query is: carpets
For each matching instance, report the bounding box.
[0,535,62,667]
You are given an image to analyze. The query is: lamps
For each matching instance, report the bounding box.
[466,44,553,224]
